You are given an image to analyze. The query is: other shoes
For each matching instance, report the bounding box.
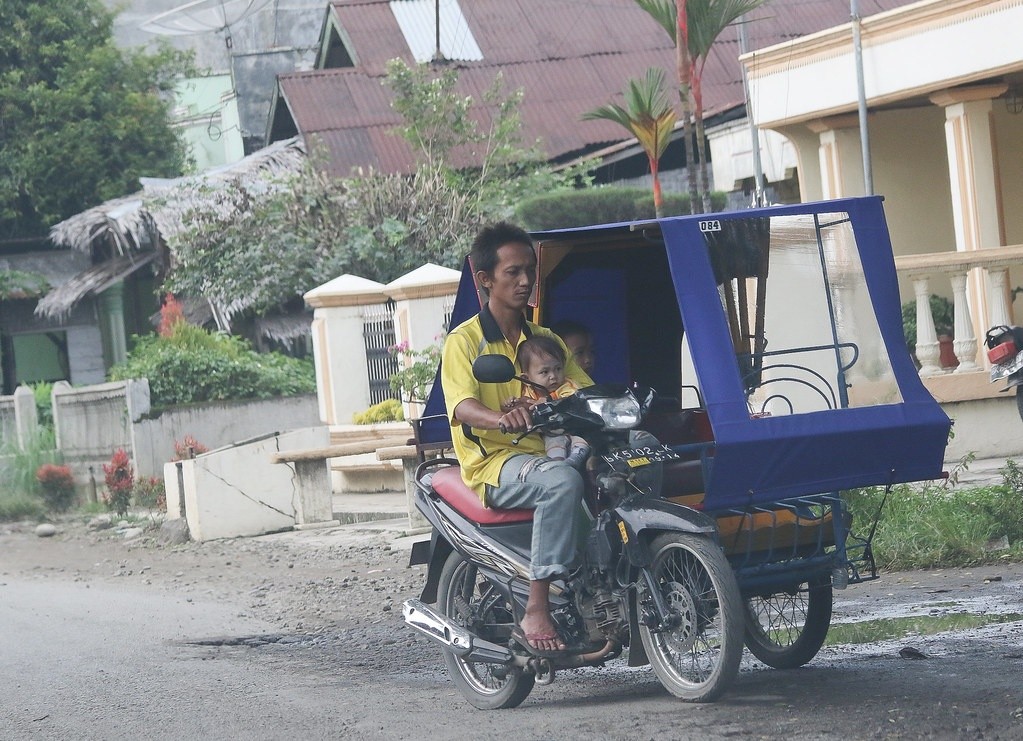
[564,455,581,470]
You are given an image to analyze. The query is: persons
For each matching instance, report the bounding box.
[556,320,618,504]
[441,221,594,650]
[500,336,590,469]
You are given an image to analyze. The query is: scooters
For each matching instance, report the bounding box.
[982,286,1023,426]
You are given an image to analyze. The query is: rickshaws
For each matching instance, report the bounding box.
[399,198,958,712]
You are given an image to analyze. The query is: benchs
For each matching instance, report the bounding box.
[617,382,714,460]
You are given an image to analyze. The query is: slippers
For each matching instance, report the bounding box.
[511,624,570,659]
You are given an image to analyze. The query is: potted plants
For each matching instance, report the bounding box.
[900,295,956,372]
